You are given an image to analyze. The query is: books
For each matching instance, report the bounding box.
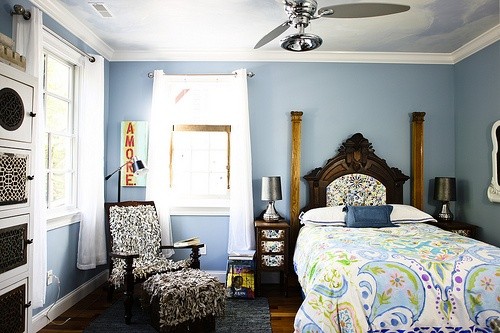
[226,266,255,298]
[229,250,256,260]
[173,237,200,248]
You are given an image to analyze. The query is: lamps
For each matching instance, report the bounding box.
[105,157,145,181]
[433,176,457,222]
[261,176,283,223]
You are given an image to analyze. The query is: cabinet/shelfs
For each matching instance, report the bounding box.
[0,0,109,333]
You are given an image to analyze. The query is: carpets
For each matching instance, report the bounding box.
[214,297,272,333]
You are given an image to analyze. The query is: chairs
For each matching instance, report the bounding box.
[103,200,204,325]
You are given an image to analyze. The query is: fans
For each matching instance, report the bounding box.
[253,0,411,53]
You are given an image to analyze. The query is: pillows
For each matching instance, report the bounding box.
[298,204,438,226]
[346,206,399,228]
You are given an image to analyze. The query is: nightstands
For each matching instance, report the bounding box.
[427,219,481,242]
[255,220,290,297]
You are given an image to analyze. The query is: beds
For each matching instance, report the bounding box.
[294,133,500,333]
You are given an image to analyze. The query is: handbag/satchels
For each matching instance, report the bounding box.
[342,205,399,227]
[225,260,255,300]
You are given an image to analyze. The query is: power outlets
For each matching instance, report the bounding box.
[48,270,53,285]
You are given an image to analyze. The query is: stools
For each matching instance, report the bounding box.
[143,268,228,333]
